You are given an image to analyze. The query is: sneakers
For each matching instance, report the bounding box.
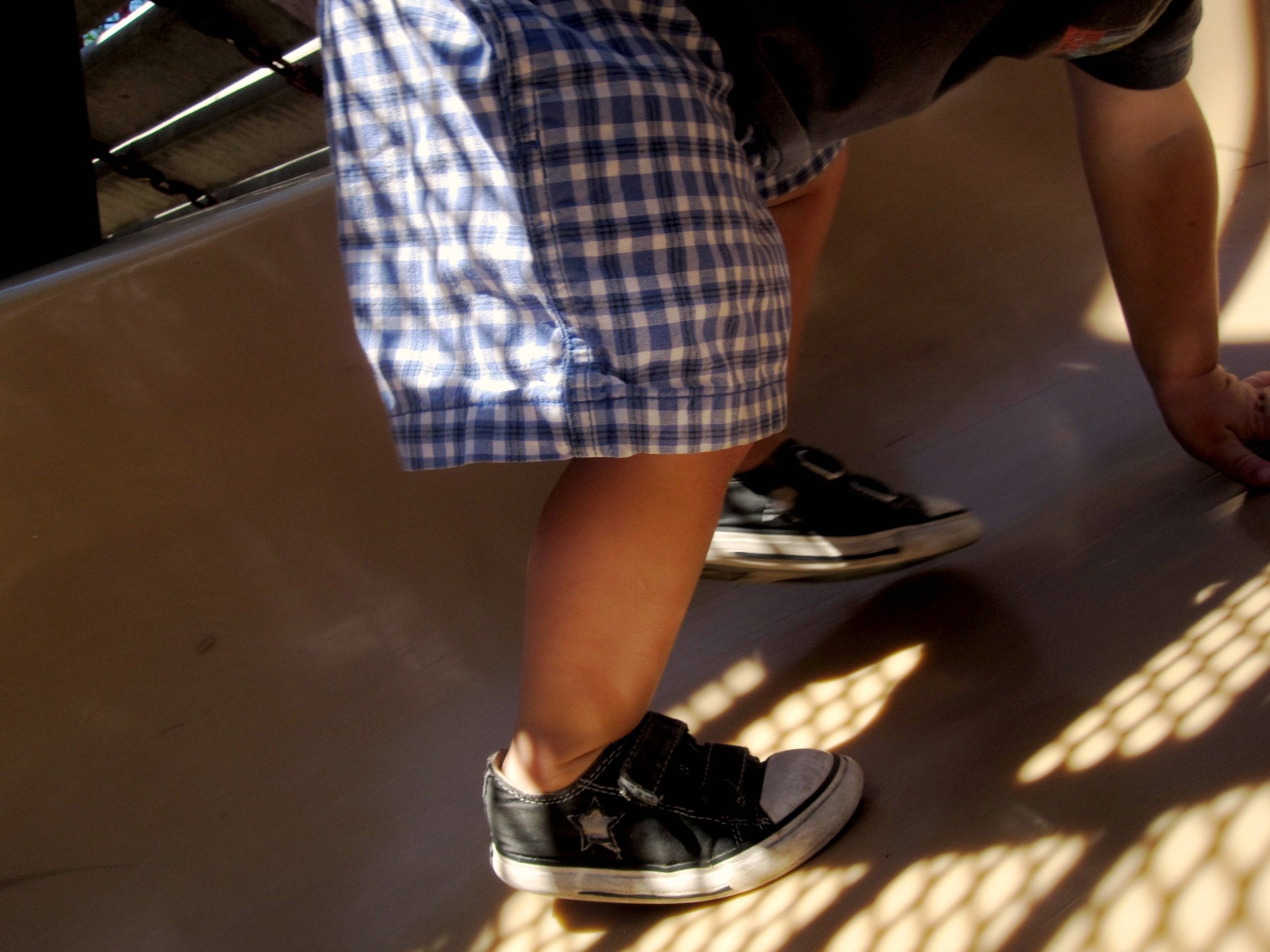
[480,709,862,903]
[703,433,985,575]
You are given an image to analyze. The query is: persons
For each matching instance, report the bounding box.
[316,0,1270,905]
